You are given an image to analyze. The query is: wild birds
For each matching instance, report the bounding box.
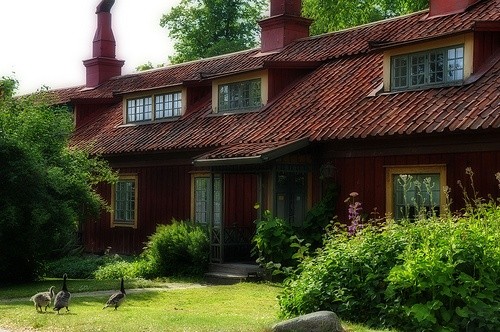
[52,273,71,316]
[29,286,56,314]
[101,277,128,311]
